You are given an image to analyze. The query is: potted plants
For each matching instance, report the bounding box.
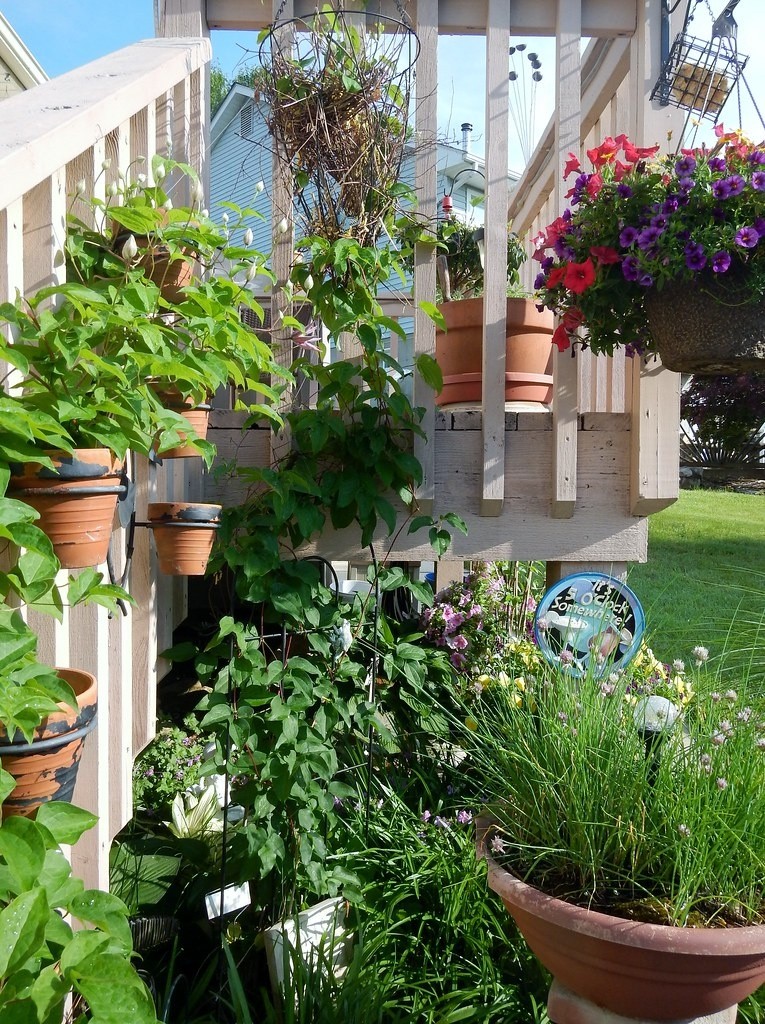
[9,1,763,1024]
